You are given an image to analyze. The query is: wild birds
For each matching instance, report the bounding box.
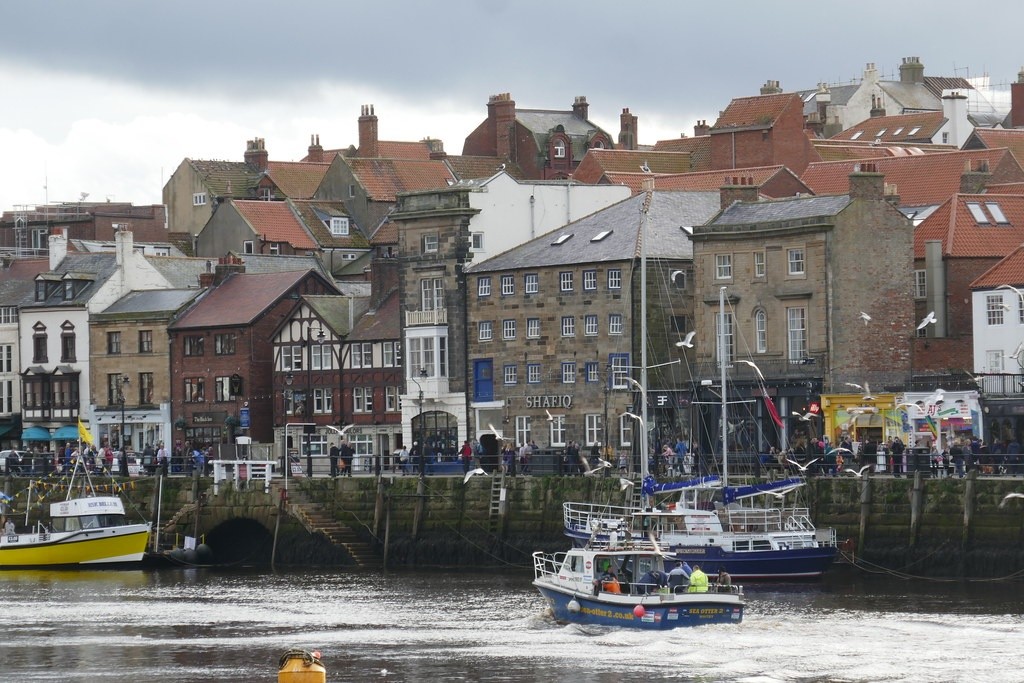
[962,368,985,385]
[648,532,681,562]
[792,380,945,456]
[998,341,1023,359]
[544,409,558,425]
[617,411,643,425]
[675,332,697,348]
[488,423,503,441]
[616,377,646,398]
[463,468,488,485]
[916,311,937,330]
[325,424,354,436]
[856,310,872,326]
[787,458,820,472]
[999,493,1024,508]
[581,457,634,491]
[993,303,1011,312]
[843,464,872,480]
[757,486,797,500]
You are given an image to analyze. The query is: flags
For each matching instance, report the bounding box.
[761,390,783,427]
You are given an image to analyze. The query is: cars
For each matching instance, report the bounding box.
[0,450,32,474]
[109,450,149,476]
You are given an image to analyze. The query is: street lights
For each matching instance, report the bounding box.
[795,356,817,476]
[116,374,130,477]
[412,367,428,476]
[274,371,294,476]
[596,363,613,476]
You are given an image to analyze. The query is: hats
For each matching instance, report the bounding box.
[718,566,727,572]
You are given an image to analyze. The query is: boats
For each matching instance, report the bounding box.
[0,442,156,566]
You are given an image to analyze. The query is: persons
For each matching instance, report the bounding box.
[0,429,1024,481]
[593,561,732,598]
[4,519,15,534]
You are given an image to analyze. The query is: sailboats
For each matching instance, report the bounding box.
[530,200,743,628]
[562,283,839,584]
